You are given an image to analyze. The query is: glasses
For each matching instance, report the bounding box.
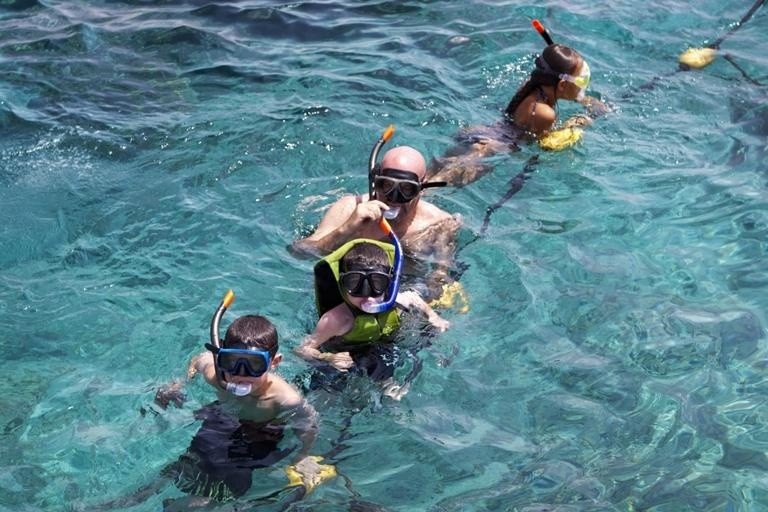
[375,174,422,204]
[216,348,269,377]
[340,271,392,298]
[569,60,591,89]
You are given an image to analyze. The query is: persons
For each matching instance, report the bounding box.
[289,146,462,304]
[428,44,608,193]
[123,315,326,512]
[291,238,450,401]
